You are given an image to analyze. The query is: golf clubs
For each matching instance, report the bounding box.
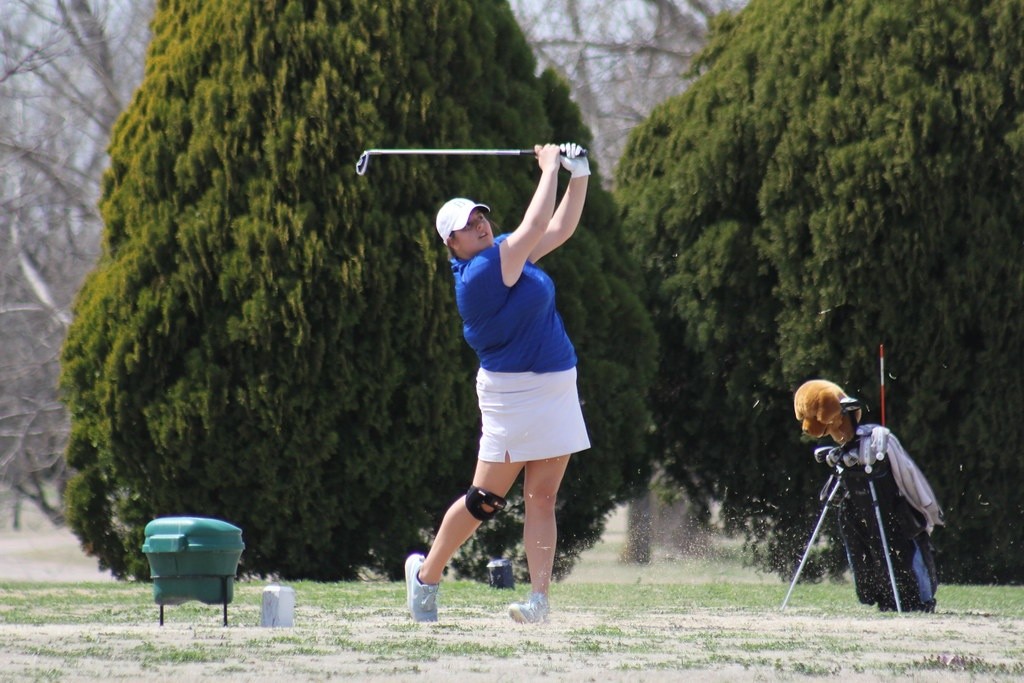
[814,446,859,508]
[356,146,589,175]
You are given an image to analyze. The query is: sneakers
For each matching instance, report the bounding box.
[404,553,441,621]
[509,590,548,622]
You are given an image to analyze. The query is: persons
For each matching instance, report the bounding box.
[405,141,592,626]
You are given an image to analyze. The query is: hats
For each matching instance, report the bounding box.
[435,198,490,244]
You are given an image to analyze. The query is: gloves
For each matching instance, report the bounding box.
[561,143,591,179]
